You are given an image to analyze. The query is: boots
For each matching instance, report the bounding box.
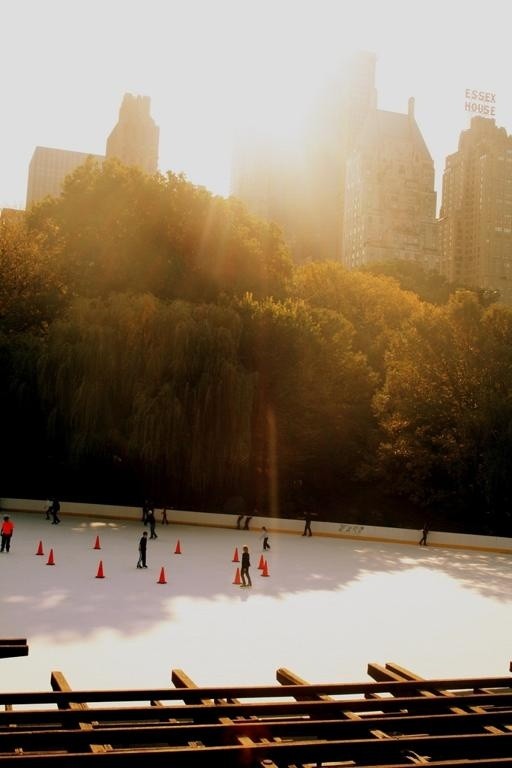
[240,583,252,587]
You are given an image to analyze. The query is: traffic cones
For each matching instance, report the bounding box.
[47,548,56,566]
[232,545,240,563]
[261,561,270,578]
[35,540,46,556]
[173,538,182,555]
[233,566,242,586]
[257,554,264,570]
[92,536,101,551]
[156,566,167,585]
[95,560,104,579]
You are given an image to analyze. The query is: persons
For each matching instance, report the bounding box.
[142,494,168,539]
[240,546,252,587]
[258,527,270,550]
[302,511,312,536]
[419,521,433,546]
[236,515,244,529]
[45,495,61,525]
[0,516,14,552]
[137,532,148,568]
[243,516,252,530]
